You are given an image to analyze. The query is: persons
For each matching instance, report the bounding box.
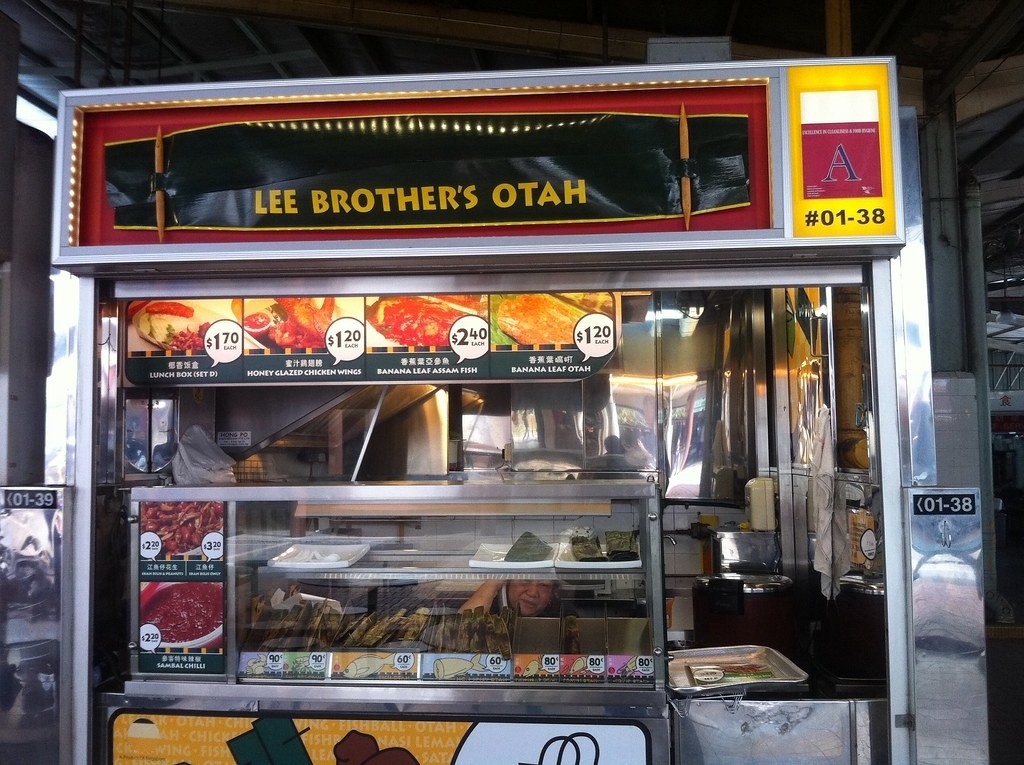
[522,409,536,441]
[511,410,520,427]
[603,435,626,456]
[454,578,579,618]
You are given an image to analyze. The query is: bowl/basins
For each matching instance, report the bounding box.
[245,307,273,343]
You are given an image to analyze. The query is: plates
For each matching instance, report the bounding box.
[267,544,370,568]
[470,542,558,569]
[555,542,643,569]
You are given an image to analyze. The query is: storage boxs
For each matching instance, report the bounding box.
[237,584,342,680]
[423,603,515,682]
[511,602,560,682]
[329,597,439,682]
[561,601,606,679]
[605,599,654,679]
